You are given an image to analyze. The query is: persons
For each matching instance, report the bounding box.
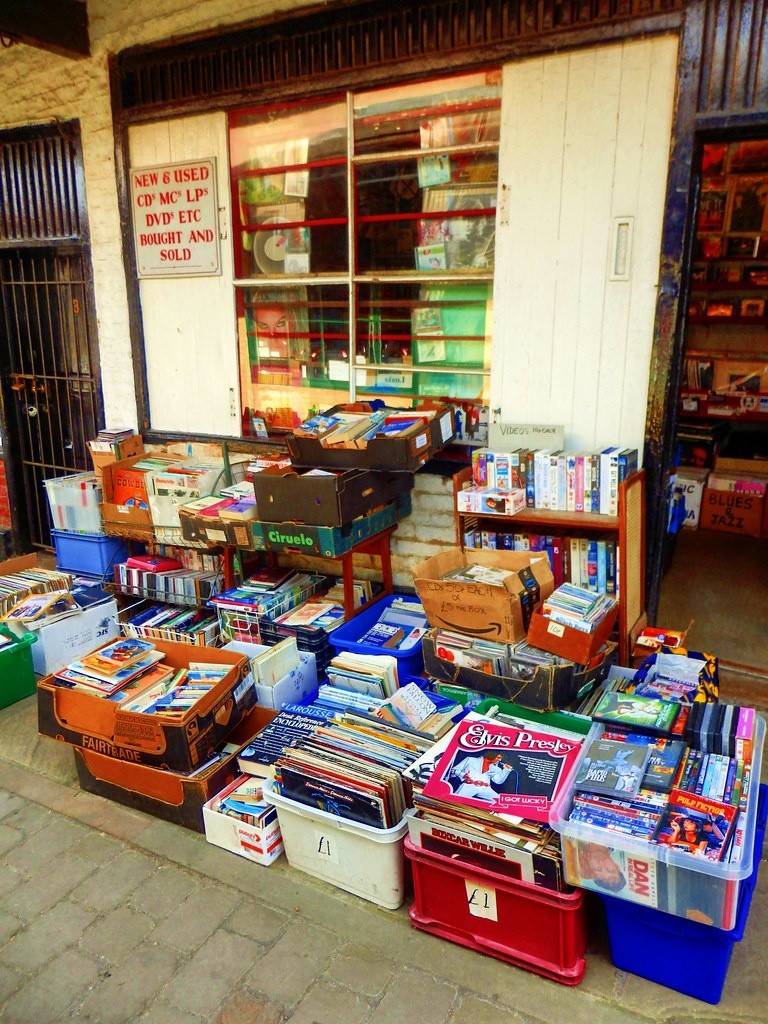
[667,816,708,855]
[251,286,301,358]
[701,813,724,856]
[684,443,709,468]
[451,751,513,802]
[429,257,443,270]
[422,157,448,182]
[486,497,506,513]
[578,840,626,894]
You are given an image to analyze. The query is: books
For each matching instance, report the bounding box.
[0,544,430,777]
[471,446,638,517]
[210,650,587,893]
[436,524,620,682]
[46,428,347,533]
[292,410,438,449]
[563,671,756,931]
[418,154,451,189]
[676,465,768,498]
[413,244,447,270]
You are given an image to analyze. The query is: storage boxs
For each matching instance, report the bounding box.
[1,391,768,1005]
